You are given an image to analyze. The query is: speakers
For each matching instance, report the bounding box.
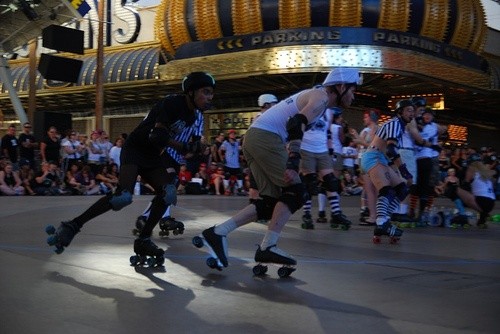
[43,24,84,54]
[37,54,83,83]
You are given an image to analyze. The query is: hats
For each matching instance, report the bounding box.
[21,160,31,168]
[364,108,381,122]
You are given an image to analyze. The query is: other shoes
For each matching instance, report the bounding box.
[14,186,118,195]
[176,188,249,196]
[359,208,372,225]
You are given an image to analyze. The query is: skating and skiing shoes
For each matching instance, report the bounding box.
[159,216,184,236]
[475,210,494,228]
[301,213,316,229]
[253,242,298,278]
[317,210,329,223]
[129,236,165,266]
[372,220,404,244]
[132,214,153,237]
[193,225,229,270]
[400,211,428,227]
[449,213,471,229]
[45,219,81,255]
[330,211,354,230]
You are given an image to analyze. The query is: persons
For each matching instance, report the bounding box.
[0,123,155,196]
[193,68,362,277]
[248,94,277,223]
[323,98,500,244]
[46,72,214,266]
[178,130,249,196]
[133,110,211,237]
[300,86,351,230]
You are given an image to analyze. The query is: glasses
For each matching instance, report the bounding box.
[24,125,32,129]
[71,132,105,138]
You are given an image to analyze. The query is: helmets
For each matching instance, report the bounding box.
[183,70,215,95]
[325,107,342,121]
[258,94,277,108]
[323,68,364,87]
[227,129,236,135]
[441,141,470,150]
[468,152,481,164]
[395,96,437,117]
